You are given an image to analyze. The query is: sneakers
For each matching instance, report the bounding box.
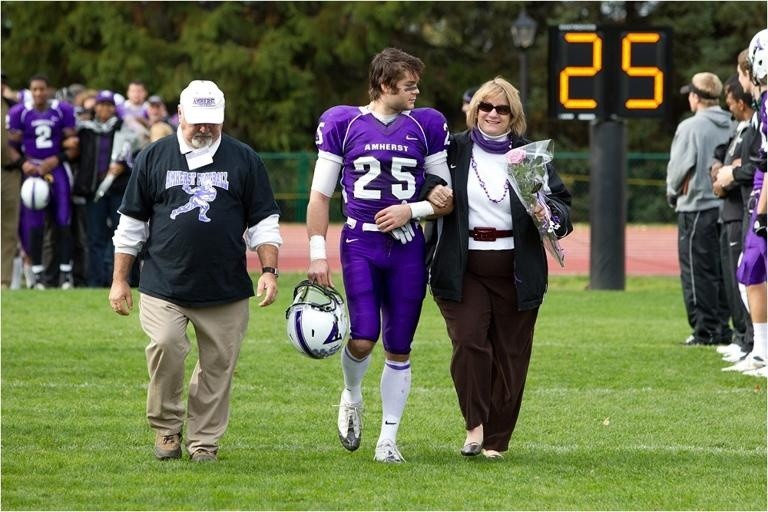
[154,432,183,462]
[723,353,761,375]
[373,440,403,464]
[337,389,363,451]
[745,363,768,384]
[189,448,218,466]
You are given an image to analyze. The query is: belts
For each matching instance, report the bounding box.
[346,216,382,233]
[468,226,513,243]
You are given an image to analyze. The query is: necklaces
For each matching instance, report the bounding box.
[462,134,515,203]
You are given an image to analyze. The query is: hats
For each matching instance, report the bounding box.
[178,78,225,127]
[681,80,719,99]
[96,89,115,106]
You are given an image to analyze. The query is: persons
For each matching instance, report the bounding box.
[721,27,768,377]
[306,46,450,465]
[0,75,73,290]
[422,77,574,460]
[722,46,762,378]
[717,80,759,365]
[130,93,169,165]
[74,91,130,286]
[109,79,283,462]
[665,73,733,346]
[120,80,148,122]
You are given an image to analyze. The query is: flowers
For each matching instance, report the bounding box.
[93,132,143,203]
[505,148,564,268]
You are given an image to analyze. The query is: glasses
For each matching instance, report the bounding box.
[478,102,512,116]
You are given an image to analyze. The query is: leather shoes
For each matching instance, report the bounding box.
[461,434,483,456]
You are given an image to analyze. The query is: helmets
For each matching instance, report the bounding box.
[285,279,349,359]
[748,28,768,84]
[21,176,51,211]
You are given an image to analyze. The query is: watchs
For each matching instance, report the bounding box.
[261,266,279,277]
